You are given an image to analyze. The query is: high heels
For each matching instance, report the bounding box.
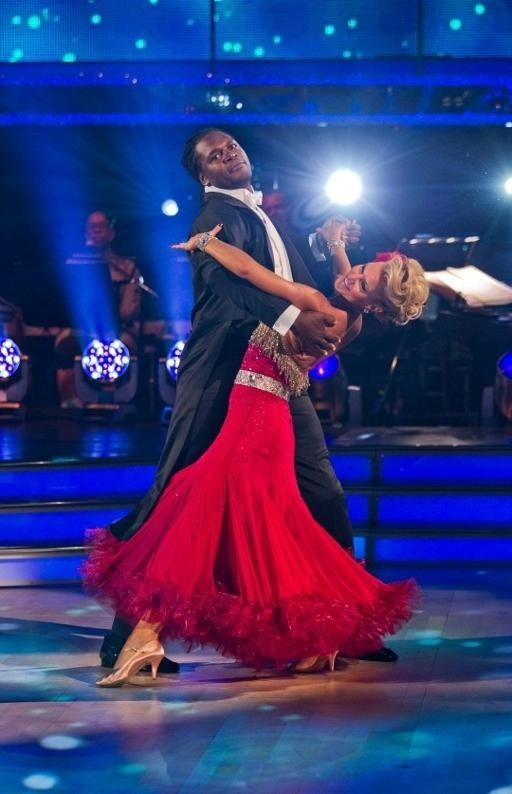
[289,649,339,674]
[96,640,164,688]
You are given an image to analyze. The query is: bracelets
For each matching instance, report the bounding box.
[198,232,216,252]
[318,235,325,252]
[326,239,346,249]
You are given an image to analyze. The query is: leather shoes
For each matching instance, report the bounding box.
[357,646,400,662]
[100,631,179,673]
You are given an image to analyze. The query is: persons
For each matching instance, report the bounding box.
[96,127,398,673]
[96,216,430,686]
[82,208,143,327]
